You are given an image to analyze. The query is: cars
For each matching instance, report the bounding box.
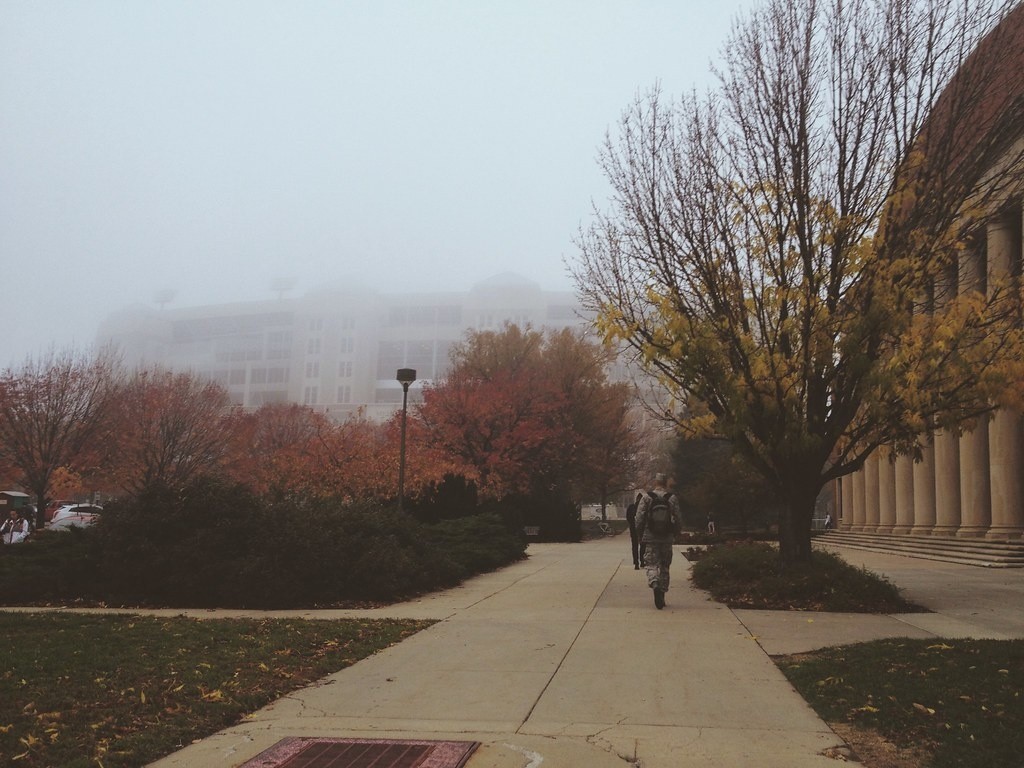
[51,503,103,531]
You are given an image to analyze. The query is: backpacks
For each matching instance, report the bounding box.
[647,491,675,534]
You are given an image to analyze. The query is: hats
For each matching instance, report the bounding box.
[654,473,667,482]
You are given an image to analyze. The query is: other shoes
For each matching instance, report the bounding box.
[641,559,644,567]
[653,581,665,609]
[634,563,640,569]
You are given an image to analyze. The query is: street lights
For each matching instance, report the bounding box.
[395,368,416,513]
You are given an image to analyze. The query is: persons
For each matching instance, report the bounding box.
[0,508,28,544]
[626,493,647,571]
[825,512,832,529]
[707,512,715,533]
[634,472,682,610]
